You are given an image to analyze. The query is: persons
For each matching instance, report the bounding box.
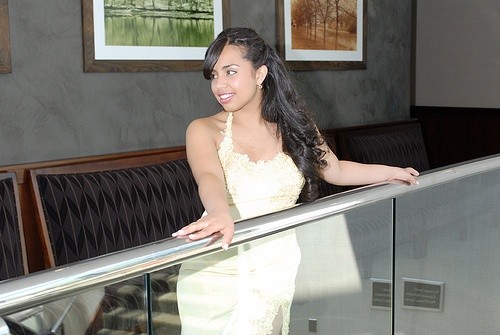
[172,27,420,335]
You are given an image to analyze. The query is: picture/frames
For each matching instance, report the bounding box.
[274,0,368,73]
[81,0,232,73]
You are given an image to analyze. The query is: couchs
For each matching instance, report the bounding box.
[0,117,439,283]
